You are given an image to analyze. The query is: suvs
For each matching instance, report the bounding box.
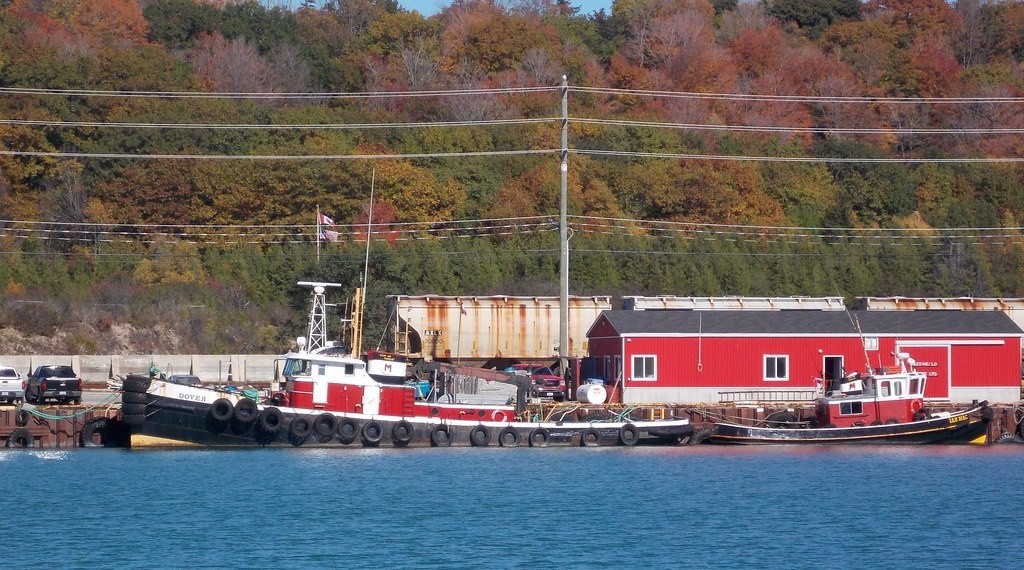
[0,366,25,405]
[511,362,566,403]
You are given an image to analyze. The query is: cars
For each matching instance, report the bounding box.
[167,375,203,390]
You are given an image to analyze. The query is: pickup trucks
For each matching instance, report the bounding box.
[26,363,83,407]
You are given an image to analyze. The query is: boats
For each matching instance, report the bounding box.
[689,351,994,447]
[119,282,696,448]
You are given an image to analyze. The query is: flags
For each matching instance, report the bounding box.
[319,214,335,229]
[319,230,339,242]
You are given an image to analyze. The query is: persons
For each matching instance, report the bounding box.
[148,362,160,376]
[291,359,300,376]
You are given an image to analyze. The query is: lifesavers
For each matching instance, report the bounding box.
[259,405,283,431]
[824,424,837,429]
[530,429,551,447]
[209,398,232,420]
[120,374,151,424]
[852,421,867,428]
[871,420,883,426]
[431,425,455,447]
[391,420,414,442]
[291,416,313,439]
[581,428,602,447]
[314,412,337,438]
[338,416,360,440]
[491,410,508,422]
[472,425,491,446]
[235,398,258,422]
[79,415,120,449]
[16,409,30,425]
[910,399,924,411]
[498,428,521,448]
[6,428,34,447]
[886,417,901,424]
[362,420,384,443]
[619,423,641,445]
